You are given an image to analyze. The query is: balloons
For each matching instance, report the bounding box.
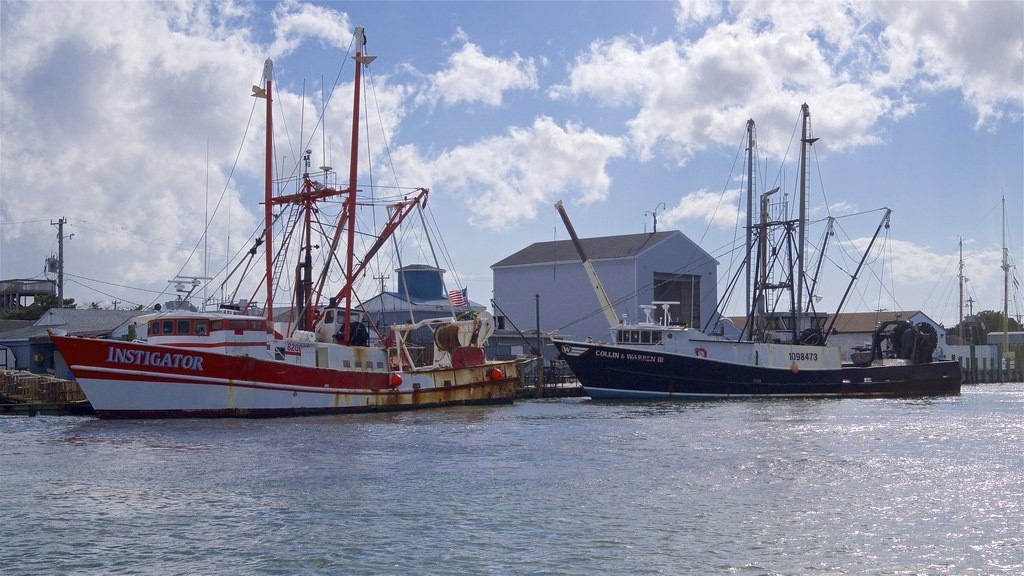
[392,374,403,386]
[492,369,503,379]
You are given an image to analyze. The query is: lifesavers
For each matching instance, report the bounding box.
[695,347,708,357]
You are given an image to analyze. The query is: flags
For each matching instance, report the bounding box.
[449,288,469,307]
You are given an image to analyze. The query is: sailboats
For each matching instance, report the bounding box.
[46,25,530,420]
[547,102,963,401]
